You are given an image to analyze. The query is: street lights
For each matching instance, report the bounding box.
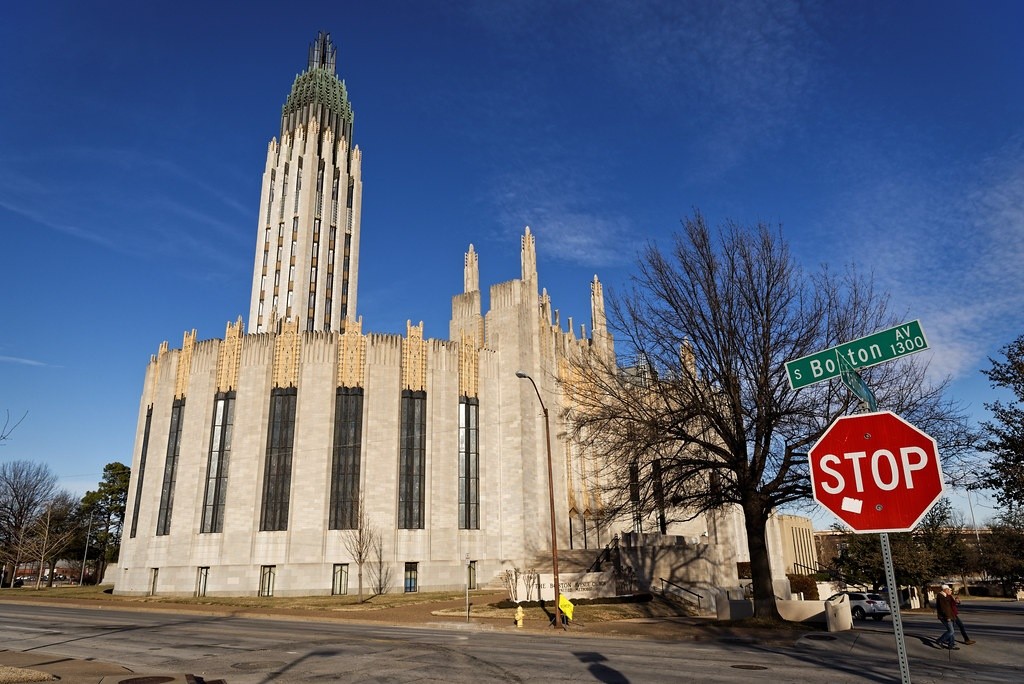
[516,372,564,628]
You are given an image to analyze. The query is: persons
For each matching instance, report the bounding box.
[936,585,960,649]
[946,587,976,645]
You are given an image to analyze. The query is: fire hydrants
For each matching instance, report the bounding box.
[514,606,526,627]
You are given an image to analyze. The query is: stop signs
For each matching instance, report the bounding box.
[805,407,945,534]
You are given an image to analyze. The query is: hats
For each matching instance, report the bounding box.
[941,584,949,589]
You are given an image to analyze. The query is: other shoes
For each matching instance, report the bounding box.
[965,640,976,645]
[936,640,944,648]
[949,646,960,651]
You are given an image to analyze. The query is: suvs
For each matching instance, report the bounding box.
[824,590,891,620]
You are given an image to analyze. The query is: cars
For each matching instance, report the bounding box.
[7,571,64,585]
